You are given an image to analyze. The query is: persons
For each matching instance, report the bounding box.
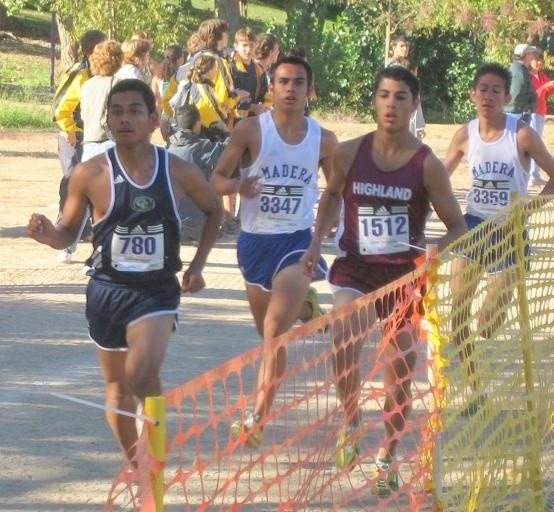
[439,59,554,419]
[404,60,427,144]
[208,55,342,451]
[387,30,413,70]
[50,18,316,264]
[295,65,475,500]
[24,78,226,480]
[502,42,537,127]
[527,47,554,186]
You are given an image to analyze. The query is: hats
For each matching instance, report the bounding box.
[514,44,536,55]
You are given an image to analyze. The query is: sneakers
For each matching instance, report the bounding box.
[336,425,360,472]
[57,250,70,262]
[305,288,329,333]
[374,453,398,498]
[231,411,263,447]
[452,386,486,415]
